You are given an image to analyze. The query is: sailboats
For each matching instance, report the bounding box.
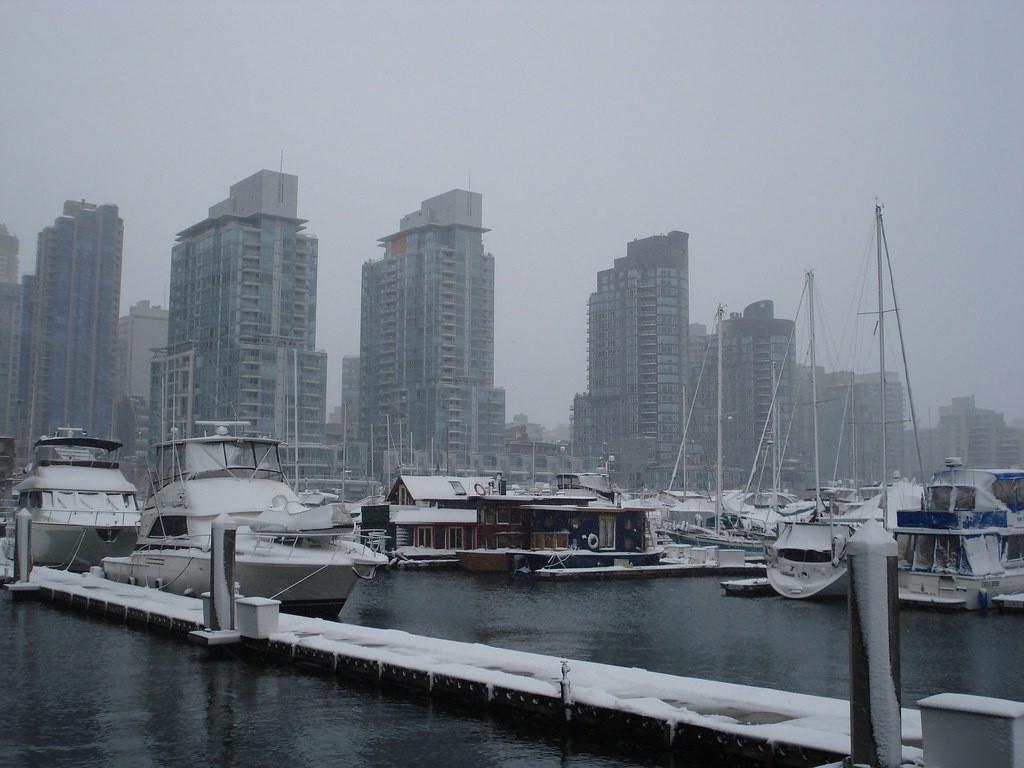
[762,185,927,605]
[283,265,879,579]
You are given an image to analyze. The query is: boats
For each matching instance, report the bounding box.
[891,454,1024,614]
[0,388,142,577]
[95,370,390,619]
